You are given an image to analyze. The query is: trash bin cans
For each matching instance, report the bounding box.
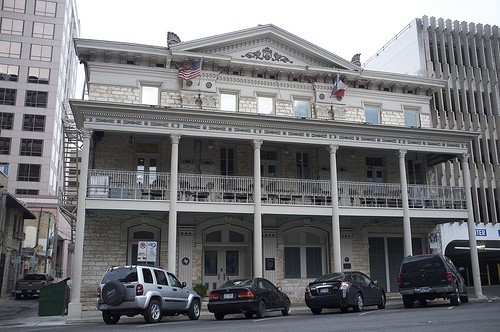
[38,277,70,317]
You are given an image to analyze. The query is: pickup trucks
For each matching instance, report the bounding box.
[15,274,55,299]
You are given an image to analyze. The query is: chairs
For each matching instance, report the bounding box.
[261,184,297,205]
[221,183,254,203]
[178,180,214,201]
[350,188,402,207]
[139,179,167,200]
[310,187,343,205]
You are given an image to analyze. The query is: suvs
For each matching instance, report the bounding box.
[95,266,202,325]
[397,254,468,308]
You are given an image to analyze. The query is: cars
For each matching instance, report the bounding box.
[305,271,386,314]
[207,277,291,321]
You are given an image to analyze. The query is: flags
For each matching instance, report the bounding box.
[330,79,350,99]
[176,60,202,81]
